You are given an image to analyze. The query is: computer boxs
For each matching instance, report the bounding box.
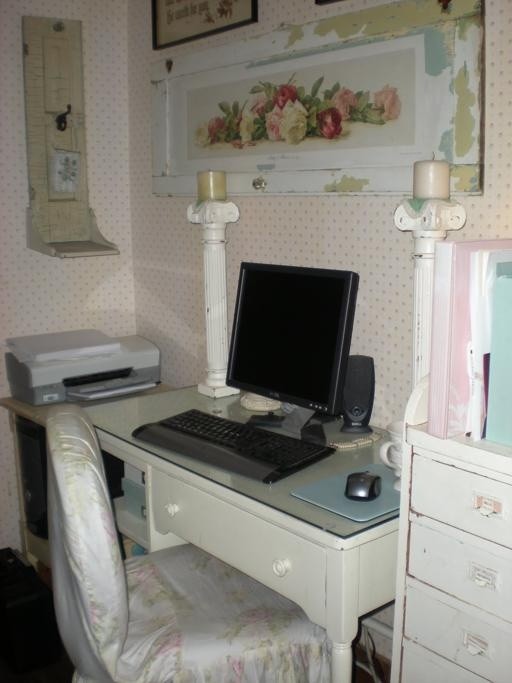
[16,415,124,540]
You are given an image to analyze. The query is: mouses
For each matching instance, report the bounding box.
[345,472,381,502]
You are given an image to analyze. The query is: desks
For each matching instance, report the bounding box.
[83,384,400,682]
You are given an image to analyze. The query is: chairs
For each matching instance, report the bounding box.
[45,404,331,681]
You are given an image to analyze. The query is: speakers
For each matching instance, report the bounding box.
[340,356,375,433]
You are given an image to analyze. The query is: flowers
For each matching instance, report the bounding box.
[193,74,402,153]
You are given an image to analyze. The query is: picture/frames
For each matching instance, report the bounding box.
[149,1,484,198]
[150,2,257,50]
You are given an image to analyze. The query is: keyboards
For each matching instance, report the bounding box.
[132,409,336,484]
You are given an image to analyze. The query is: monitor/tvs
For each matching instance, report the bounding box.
[226,262,359,416]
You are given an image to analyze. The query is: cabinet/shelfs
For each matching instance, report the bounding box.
[391,371,512,683]
[1,380,177,571]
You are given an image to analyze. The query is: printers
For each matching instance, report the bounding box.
[5,335,161,407]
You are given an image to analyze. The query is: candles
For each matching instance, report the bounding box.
[413,151,450,197]
[198,171,226,200]
